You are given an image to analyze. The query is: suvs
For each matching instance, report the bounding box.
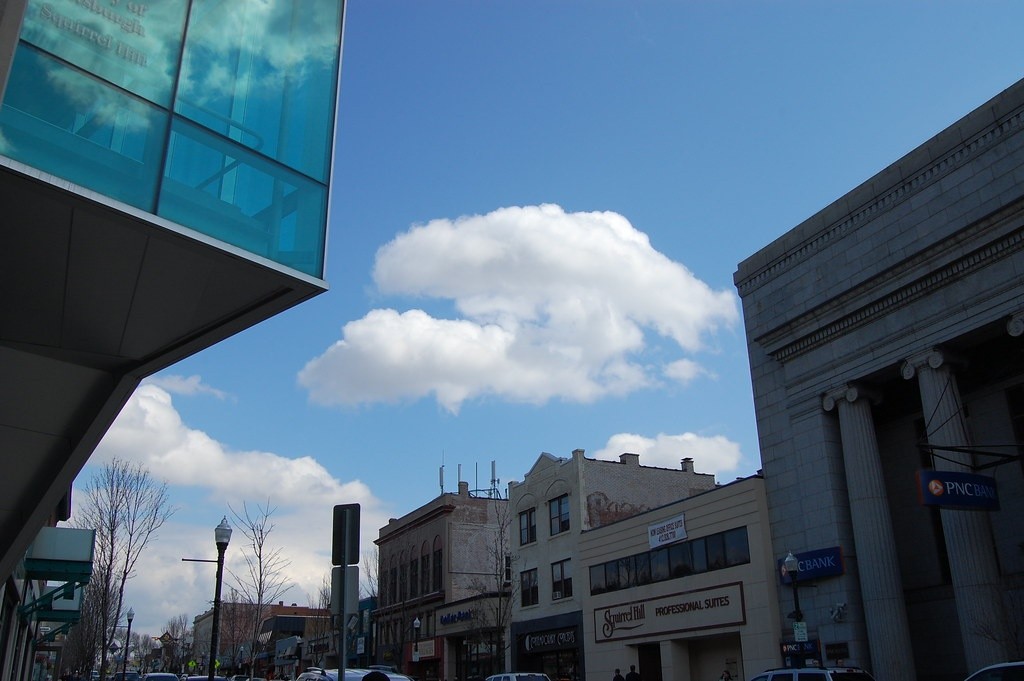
[750,666,878,681]
[180,674,230,681]
[295,665,414,681]
[486,673,551,681]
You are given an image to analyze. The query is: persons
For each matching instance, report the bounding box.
[837,658,845,667]
[626,665,641,681]
[718,670,734,681]
[813,659,822,667]
[613,669,625,681]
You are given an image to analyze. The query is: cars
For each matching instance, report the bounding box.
[962,662,1024,681]
[231,674,268,681]
[92,670,143,681]
[143,672,179,681]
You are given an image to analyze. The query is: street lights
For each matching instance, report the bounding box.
[201,651,205,675]
[206,514,233,681]
[783,550,807,668]
[413,616,421,681]
[239,646,244,675]
[121,606,135,681]
